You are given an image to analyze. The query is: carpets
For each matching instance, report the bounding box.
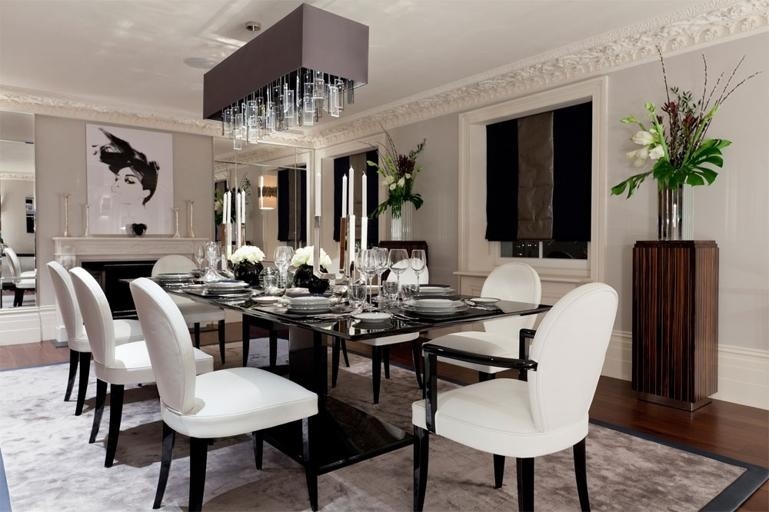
[0,335,768,512]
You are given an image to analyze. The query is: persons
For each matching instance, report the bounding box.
[91,127,160,234]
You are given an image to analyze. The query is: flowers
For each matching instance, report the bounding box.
[290,245,331,269]
[230,246,266,265]
[359,117,427,220]
[607,41,762,235]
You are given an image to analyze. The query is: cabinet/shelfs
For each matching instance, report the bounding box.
[632,241,720,413]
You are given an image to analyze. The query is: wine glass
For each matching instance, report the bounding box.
[262,244,294,295]
[195,239,222,282]
[329,246,425,309]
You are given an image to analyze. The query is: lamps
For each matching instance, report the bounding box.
[202,3,369,151]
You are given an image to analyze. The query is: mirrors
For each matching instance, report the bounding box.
[0,109,38,309]
[211,135,315,262]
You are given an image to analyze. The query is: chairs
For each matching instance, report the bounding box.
[421,260,542,397]
[47,261,146,414]
[69,265,215,469]
[411,281,620,508]
[331,258,429,404]
[4,247,35,307]
[128,276,321,512]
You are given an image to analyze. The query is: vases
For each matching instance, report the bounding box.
[655,177,684,241]
[294,265,330,295]
[234,260,265,286]
[391,202,414,240]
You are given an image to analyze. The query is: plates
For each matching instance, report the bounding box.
[252,296,281,304]
[350,322,393,329]
[156,273,194,281]
[286,296,330,314]
[402,300,469,315]
[353,313,393,323]
[415,286,455,296]
[205,280,250,294]
[470,298,500,304]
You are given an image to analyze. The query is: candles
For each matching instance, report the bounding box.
[341,163,368,220]
[222,188,246,225]
